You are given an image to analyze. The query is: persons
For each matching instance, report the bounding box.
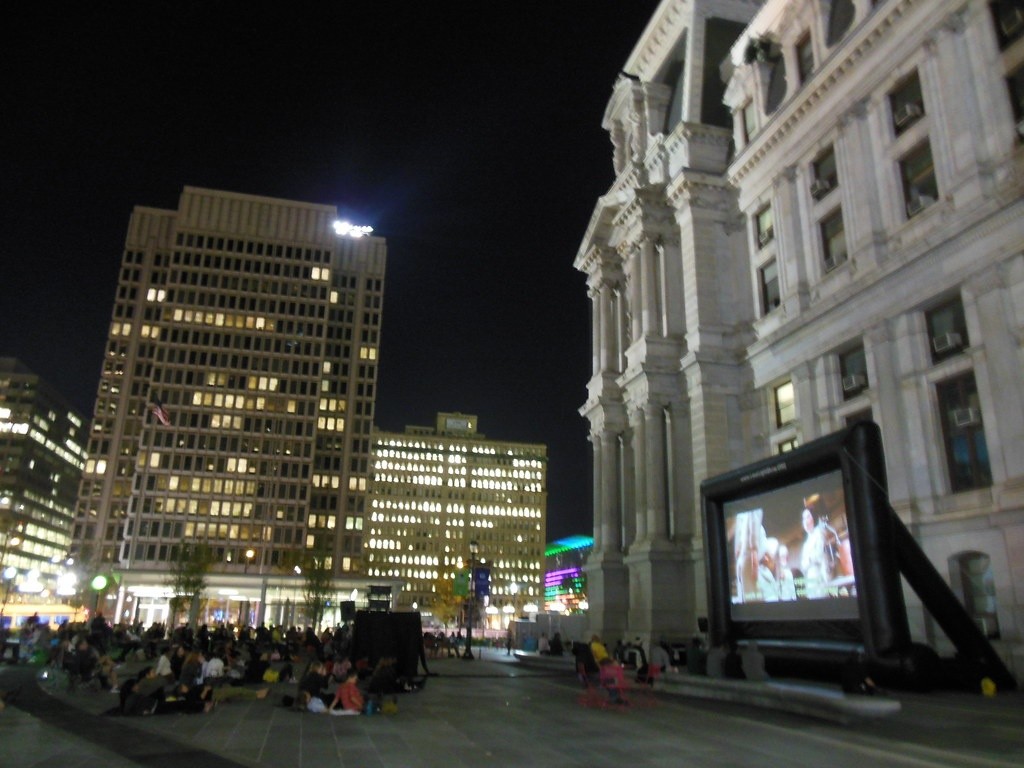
[537,632,779,706]
[734,506,831,602]
[505,628,513,657]
[0,611,461,719]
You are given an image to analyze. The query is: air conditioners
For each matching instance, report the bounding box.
[892,103,921,127]
[809,179,826,200]
[842,375,866,392]
[953,408,980,429]
[932,333,962,353]
[824,256,839,273]
[907,195,934,216]
[758,231,769,245]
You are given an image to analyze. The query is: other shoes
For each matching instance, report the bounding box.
[258,688,270,700]
[205,700,217,714]
[111,685,119,693]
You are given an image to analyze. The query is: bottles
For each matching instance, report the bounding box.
[367,700,372,716]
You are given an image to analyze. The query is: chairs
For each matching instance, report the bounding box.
[640,665,659,703]
[597,664,632,712]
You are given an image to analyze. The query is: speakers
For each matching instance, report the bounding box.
[697,617,708,632]
[340,601,355,622]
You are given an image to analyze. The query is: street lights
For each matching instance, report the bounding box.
[461,539,481,660]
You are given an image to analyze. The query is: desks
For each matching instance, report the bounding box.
[604,683,635,710]
